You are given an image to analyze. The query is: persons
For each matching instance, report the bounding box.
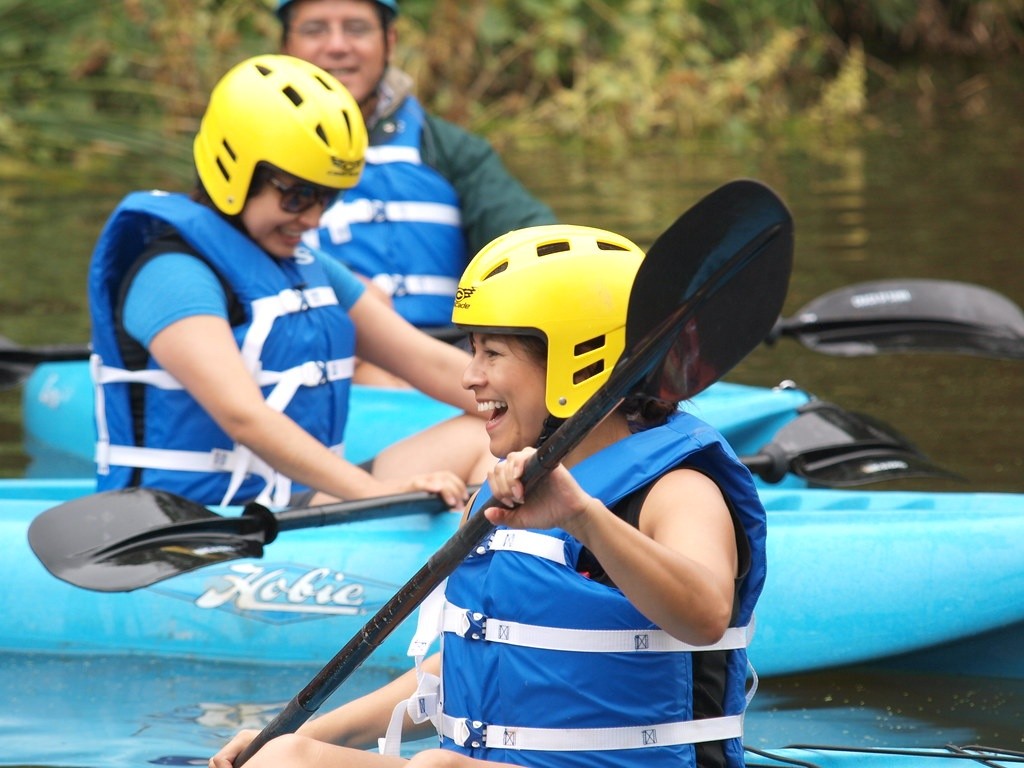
[280,1,562,390]
[207,224,770,767]
[89,53,538,510]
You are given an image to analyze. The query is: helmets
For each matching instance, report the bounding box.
[192,52,367,216]
[276,0,398,26]
[451,225,646,420]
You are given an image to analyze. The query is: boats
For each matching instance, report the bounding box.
[23,359,811,477]
[0,477,1023,678]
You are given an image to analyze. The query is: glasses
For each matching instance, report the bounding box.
[259,167,338,213]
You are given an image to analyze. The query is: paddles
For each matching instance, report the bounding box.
[760,278,1023,359]
[26,404,969,593]
[232,177,795,768]
